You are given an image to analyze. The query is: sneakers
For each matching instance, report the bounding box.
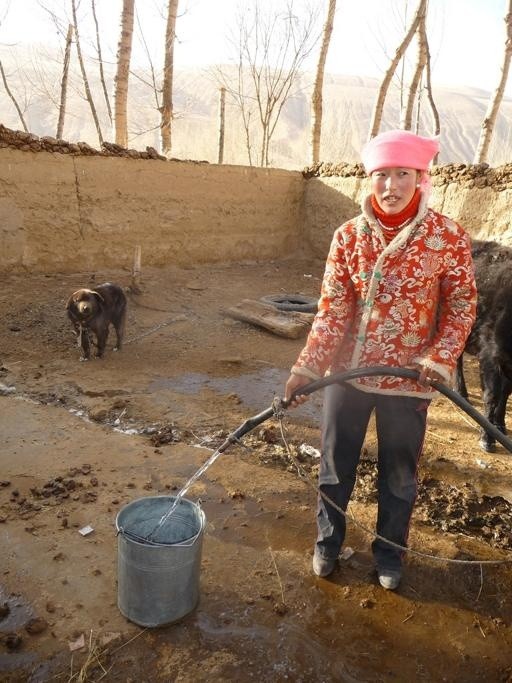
[313,543,338,577]
[375,564,401,590]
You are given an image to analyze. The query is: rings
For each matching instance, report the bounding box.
[432,378,438,381]
[425,376,431,379]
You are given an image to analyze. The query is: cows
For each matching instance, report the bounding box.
[453,239,512,453]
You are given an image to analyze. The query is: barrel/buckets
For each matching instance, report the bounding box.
[115,495,206,628]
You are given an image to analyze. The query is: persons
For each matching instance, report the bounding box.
[283,129,479,591]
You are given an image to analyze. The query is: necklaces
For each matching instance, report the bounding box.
[376,217,413,230]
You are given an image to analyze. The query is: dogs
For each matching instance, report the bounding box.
[64,282,128,361]
